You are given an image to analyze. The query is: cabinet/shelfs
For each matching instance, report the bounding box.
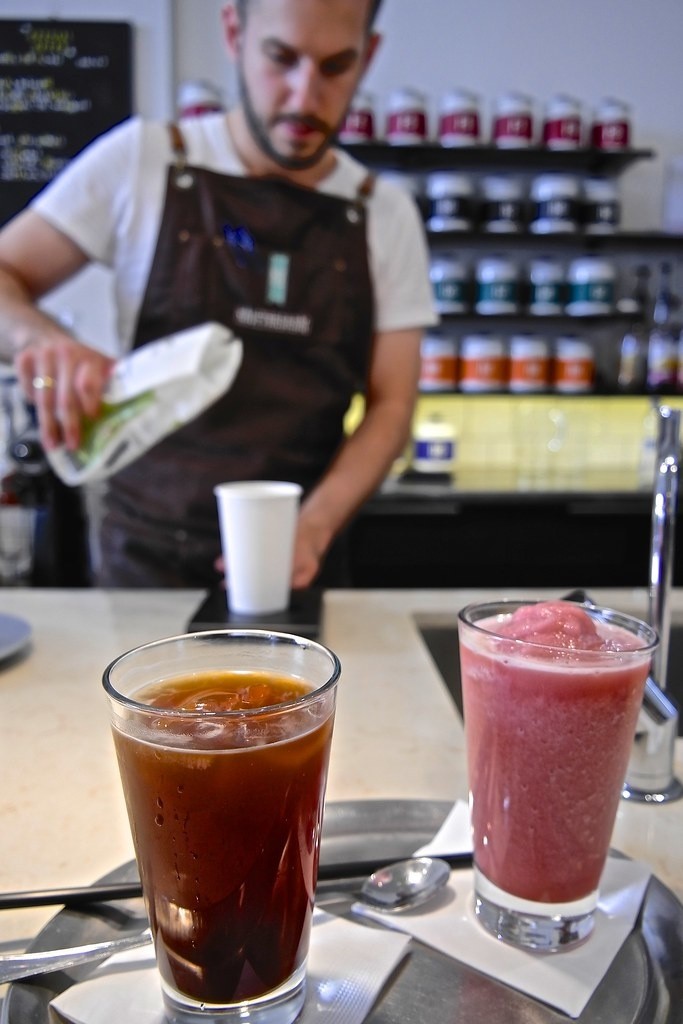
[341,498,682,589]
[335,145,682,397]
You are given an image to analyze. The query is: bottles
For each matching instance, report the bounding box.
[175,80,220,121]
[329,91,683,395]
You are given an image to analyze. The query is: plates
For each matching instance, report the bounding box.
[0,611,33,662]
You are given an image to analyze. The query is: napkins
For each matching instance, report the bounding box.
[50,907,415,1024]
[352,799,651,1019]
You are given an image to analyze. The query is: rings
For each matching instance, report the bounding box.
[33,375,57,389]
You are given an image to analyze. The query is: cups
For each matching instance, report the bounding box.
[103,627,341,1023]
[214,479,302,616]
[454,595,663,949]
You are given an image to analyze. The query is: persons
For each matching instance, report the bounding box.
[0,0,441,596]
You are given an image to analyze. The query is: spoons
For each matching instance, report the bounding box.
[0,857,453,987]
